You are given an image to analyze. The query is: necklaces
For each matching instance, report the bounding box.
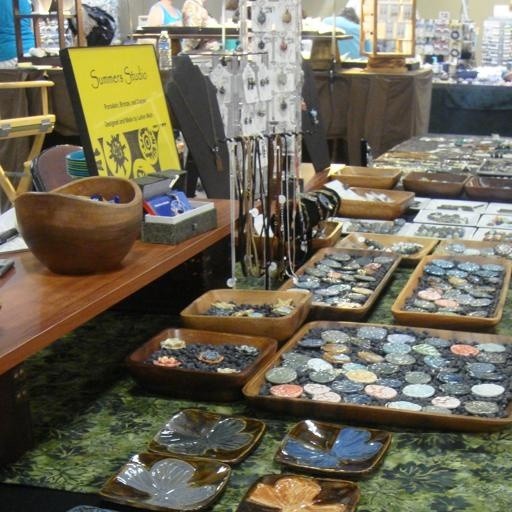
[225,130,338,288]
[302,63,319,125]
[167,71,224,172]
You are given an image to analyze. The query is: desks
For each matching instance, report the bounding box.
[0,65,85,188]
[431,80,512,136]
[309,63,432,165]
[0,200,253,378]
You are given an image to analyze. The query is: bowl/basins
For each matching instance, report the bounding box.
[15,175,144,274]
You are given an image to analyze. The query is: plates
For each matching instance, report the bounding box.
[390,256,510,329]
[277,247,403,316]
[325,196,511,255]
[65,150,90,180]
[95,409,391,512]
[181,288,311,340]
[243,320,512,429]
[125,328,278,395]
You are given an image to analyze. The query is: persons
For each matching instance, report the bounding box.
[322,8,371,58]
[0,0,36,62]
[180,0,220,56]
[148,0,183,28]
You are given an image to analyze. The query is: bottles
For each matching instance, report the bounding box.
[158,30,172,70]
